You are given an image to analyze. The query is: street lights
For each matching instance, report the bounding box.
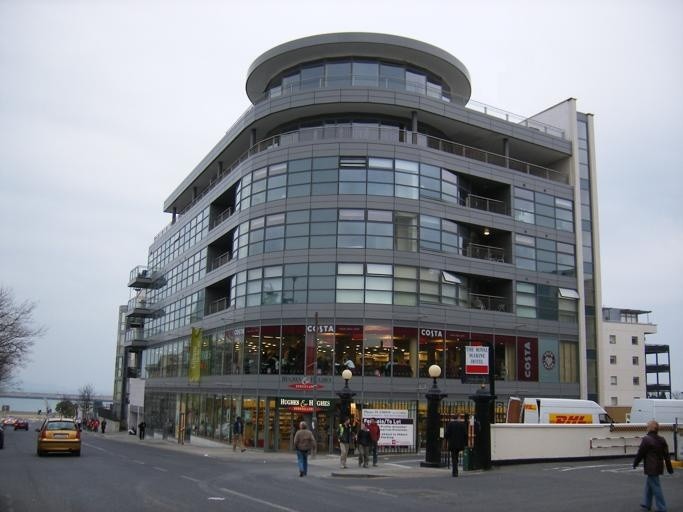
[427,364,445,396]
[337,369,357,393]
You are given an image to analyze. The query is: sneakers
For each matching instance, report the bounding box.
[340,465,345,468]
[300,471,303,476]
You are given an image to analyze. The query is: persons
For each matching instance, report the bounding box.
[336,417,356,469]
[292,419,317,477]
[231,415,246,452]
[632,420,674,512]
[76,417,99,432]
[354,421,374,468]
[444,411,469,478]
[100,418,107,434]
[138,420,146,440]
[318,354,357,376]
[260,347,292,373]
[367,418,380,467]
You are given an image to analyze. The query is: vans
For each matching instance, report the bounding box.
[506,395,618,424]
[630,398,682,424]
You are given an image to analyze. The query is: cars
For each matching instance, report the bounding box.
[13,418,29,430]
[34,417,82,457]
[2,415,17,427]
